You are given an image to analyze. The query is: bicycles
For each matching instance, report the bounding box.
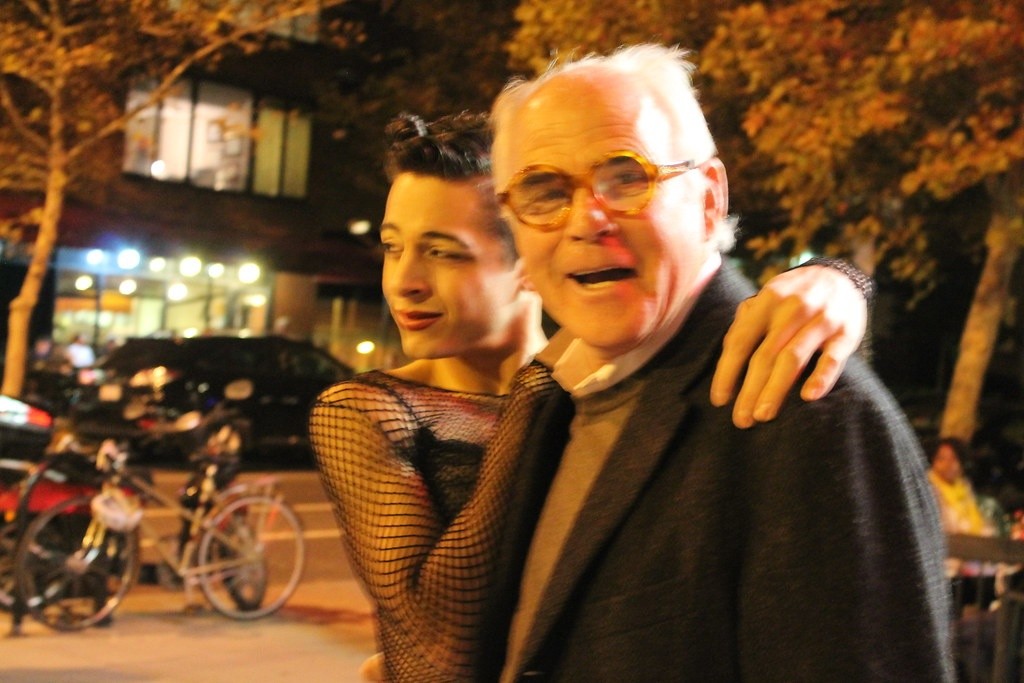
[14,461,305,632]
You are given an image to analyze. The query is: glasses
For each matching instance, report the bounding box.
[493,151,696,232]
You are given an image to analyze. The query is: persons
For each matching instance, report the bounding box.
[359,41,957,683]
[306,108,876,683]
[924,438,1001,618]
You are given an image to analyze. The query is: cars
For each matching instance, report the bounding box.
[70,333,366,473]
[0,392,51,484]
